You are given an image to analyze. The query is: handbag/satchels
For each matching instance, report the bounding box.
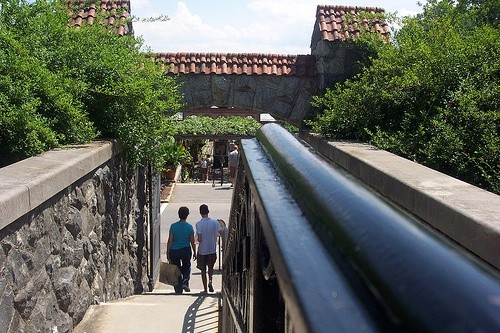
[159,257,180,286]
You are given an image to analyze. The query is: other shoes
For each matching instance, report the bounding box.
[200,291,207,294]
[208,283,214,292]
[176,292,182,295]
[182,285,190,292]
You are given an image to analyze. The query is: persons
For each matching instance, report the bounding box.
[205,154,213,179]
[167,207,197,295]
[201,157,208,183]
[196,204,224,295]
[228,144,239,187]
